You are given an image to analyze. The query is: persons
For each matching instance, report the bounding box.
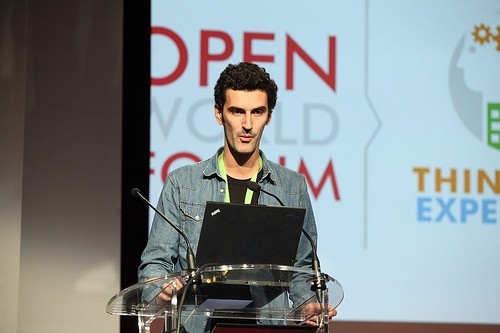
[137,61,338,333]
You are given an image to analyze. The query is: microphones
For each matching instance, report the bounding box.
[245,181,318,270]
[130,188,196,269]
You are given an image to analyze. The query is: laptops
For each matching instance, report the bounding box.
[168,201,307,308]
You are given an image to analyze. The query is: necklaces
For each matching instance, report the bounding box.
[218,150,263,206]
